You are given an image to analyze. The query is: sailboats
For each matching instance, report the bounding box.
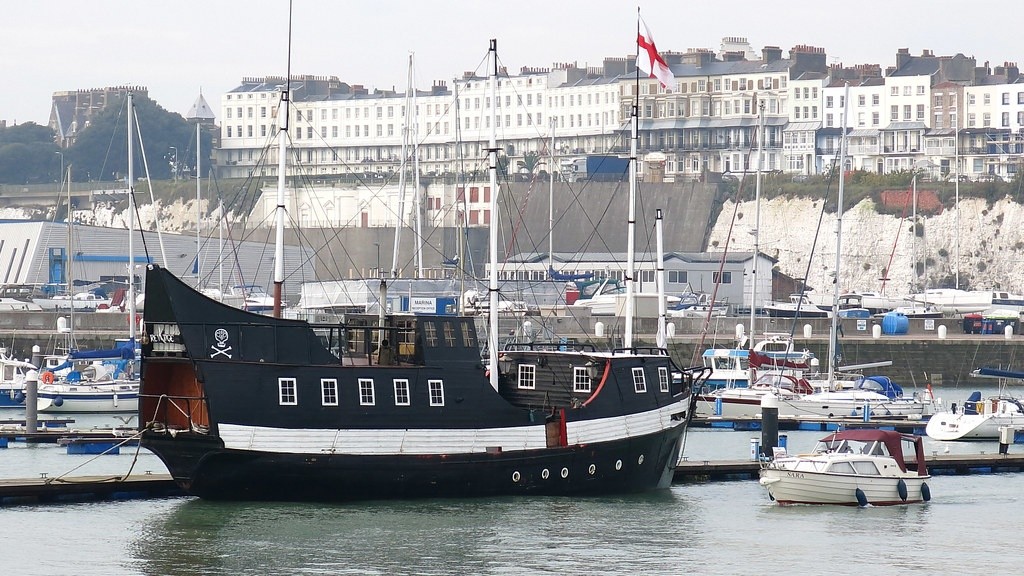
[0,0,1024,498]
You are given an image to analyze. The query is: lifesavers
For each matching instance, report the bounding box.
[127,313,140,326]
[42,371,54,384]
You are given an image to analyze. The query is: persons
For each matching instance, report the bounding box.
[924,384,933,402]
[837,313,843,338]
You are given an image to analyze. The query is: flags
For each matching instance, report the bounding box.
[635,16,675,89]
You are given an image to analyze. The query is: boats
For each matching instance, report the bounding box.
[759,428,932,504]
